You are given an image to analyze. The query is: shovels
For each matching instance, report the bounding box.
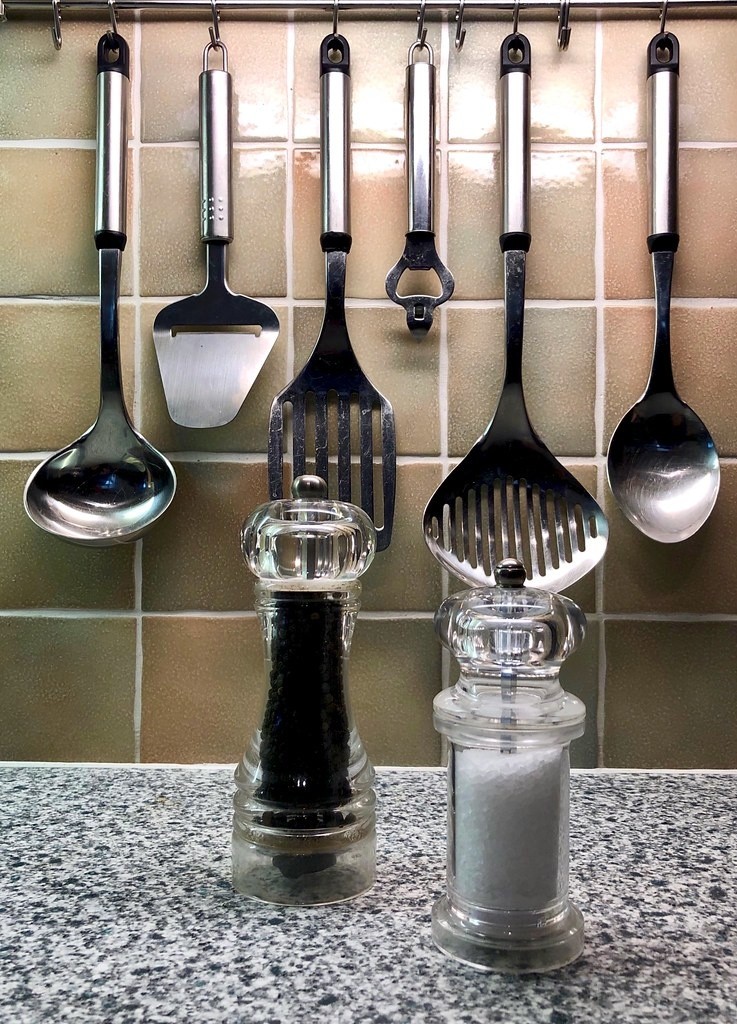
[267,33,396,553]
[153,41,281,429]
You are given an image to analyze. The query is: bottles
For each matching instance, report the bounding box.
[431,558,588,971]
[232,475,378,906]
